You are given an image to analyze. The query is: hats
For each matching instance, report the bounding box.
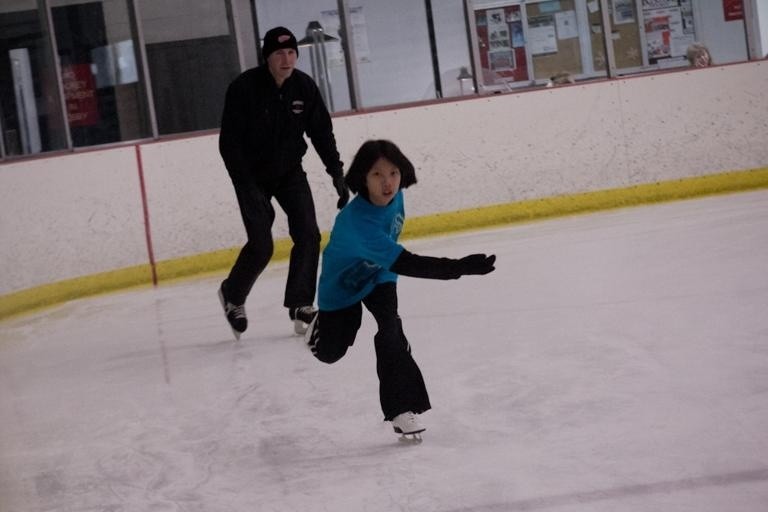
[261,26,299,57]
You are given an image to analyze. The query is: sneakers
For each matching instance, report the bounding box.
[218,288,248,332]
[392,410,426,434]
[288,305,317,324]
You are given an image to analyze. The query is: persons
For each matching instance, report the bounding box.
[303,137,499,436]
[214,23,350,335]
[684,41,714,68]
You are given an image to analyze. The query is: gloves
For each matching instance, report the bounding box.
[459,253,496,276]
[333,176,349,209]
